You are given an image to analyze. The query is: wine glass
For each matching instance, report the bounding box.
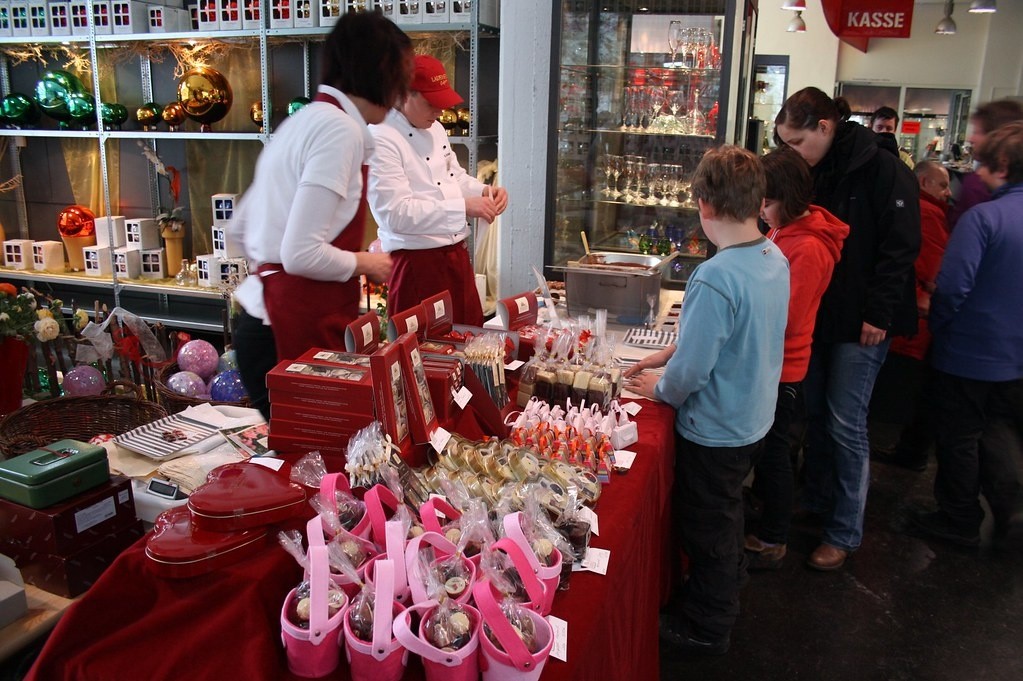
[619,86,684,134]
[667,20,720,70]
[601,153,695,208]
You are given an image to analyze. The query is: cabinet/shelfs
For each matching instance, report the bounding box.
[2,22,499,330]
[545,1,759,296]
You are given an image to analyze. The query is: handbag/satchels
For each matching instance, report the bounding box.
[279,472,562,681]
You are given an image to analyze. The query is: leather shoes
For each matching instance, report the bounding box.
[809,542,851,570]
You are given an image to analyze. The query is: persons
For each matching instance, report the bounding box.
[744,85,1023,569]
[366,55,508,328]
[623,145,790,654]
[224,11,394,420]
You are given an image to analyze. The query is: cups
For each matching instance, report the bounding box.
[61,235,95,270]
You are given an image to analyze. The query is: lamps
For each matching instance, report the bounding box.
[784,12,806,33]
[936,2,958,34]
[781,0,807,10]
[969,0,998,13]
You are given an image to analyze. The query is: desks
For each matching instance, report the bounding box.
[35,290,675,681]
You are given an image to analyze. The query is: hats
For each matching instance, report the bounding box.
[407,55,465,110]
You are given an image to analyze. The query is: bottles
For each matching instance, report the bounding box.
[174,259,197,287]
[682,89,706,135]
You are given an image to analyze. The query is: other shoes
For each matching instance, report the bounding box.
[742,536,787,573]
[664,619,731,655]
[872,444,929,470]
[905,504,981,542]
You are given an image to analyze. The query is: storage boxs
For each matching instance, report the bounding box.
[3,238,64,272]
[0,440,139,596]
[192,194,250,288]
[266,323,595,470]
[86,217,167,283]
[1,2,470,24]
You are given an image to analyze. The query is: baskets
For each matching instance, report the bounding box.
[155,353,257,414]
[0,382,162,457]
